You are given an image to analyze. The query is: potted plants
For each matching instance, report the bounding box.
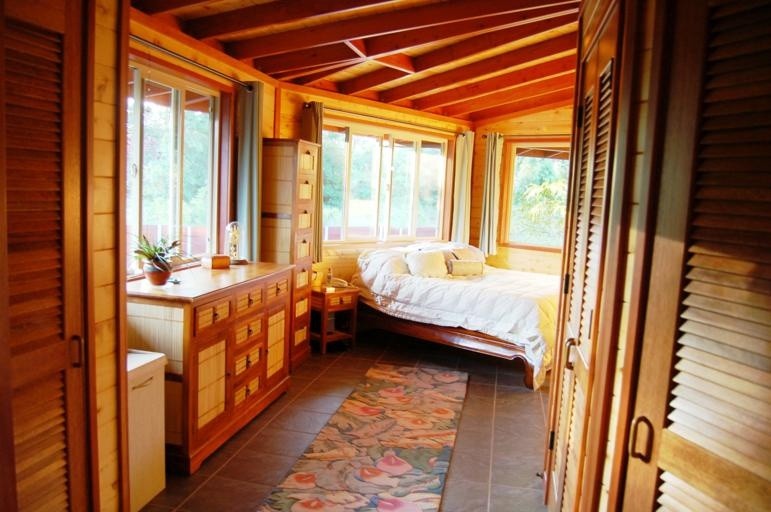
[134,234,182,286]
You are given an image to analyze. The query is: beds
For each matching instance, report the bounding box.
[348,240,562,392]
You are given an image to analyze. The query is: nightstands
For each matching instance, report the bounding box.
[310,283,360,354]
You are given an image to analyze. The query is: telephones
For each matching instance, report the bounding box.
[330,278,348,287]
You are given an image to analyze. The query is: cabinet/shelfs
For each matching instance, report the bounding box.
[125,347,168,512]
[261,138,324,377]
[127,270,294,476]
[2,2,101,512]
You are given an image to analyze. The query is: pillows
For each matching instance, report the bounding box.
[365,241,488,278]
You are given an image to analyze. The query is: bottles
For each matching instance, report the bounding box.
[326,268,332,287]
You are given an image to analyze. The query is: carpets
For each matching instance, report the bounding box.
[249,344,473,512]
